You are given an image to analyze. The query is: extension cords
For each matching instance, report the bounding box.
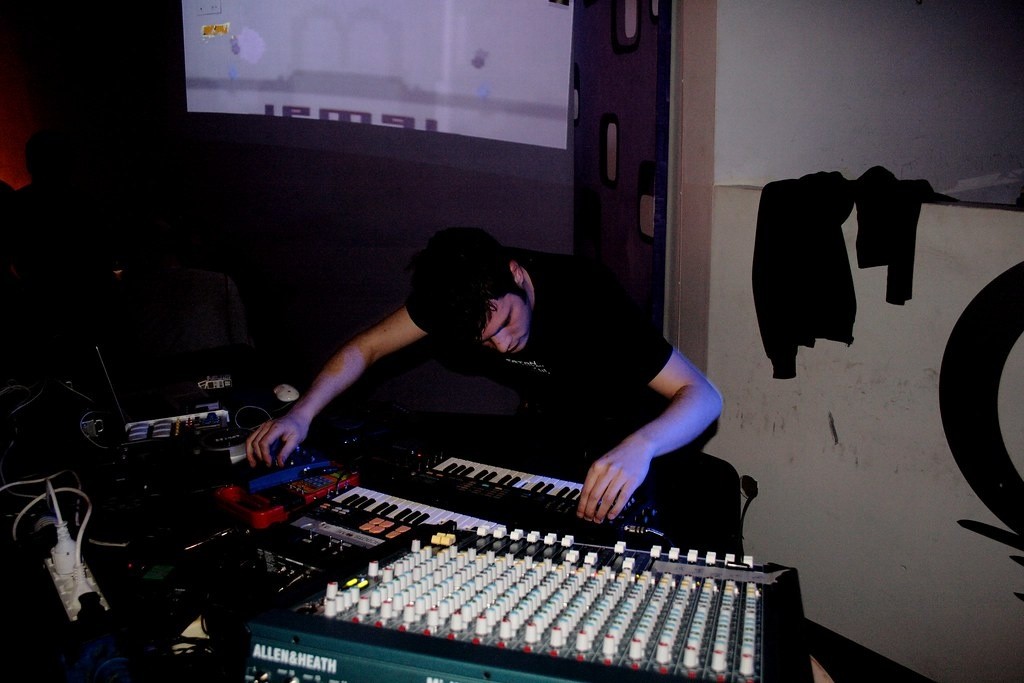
[44,553,110,622]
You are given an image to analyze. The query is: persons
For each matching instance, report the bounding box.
[246,226,721,524]
[0,128,255,353]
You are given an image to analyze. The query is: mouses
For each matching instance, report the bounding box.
[273,384,300,401]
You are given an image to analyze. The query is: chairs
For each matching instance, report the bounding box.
[700,454,746,559]
